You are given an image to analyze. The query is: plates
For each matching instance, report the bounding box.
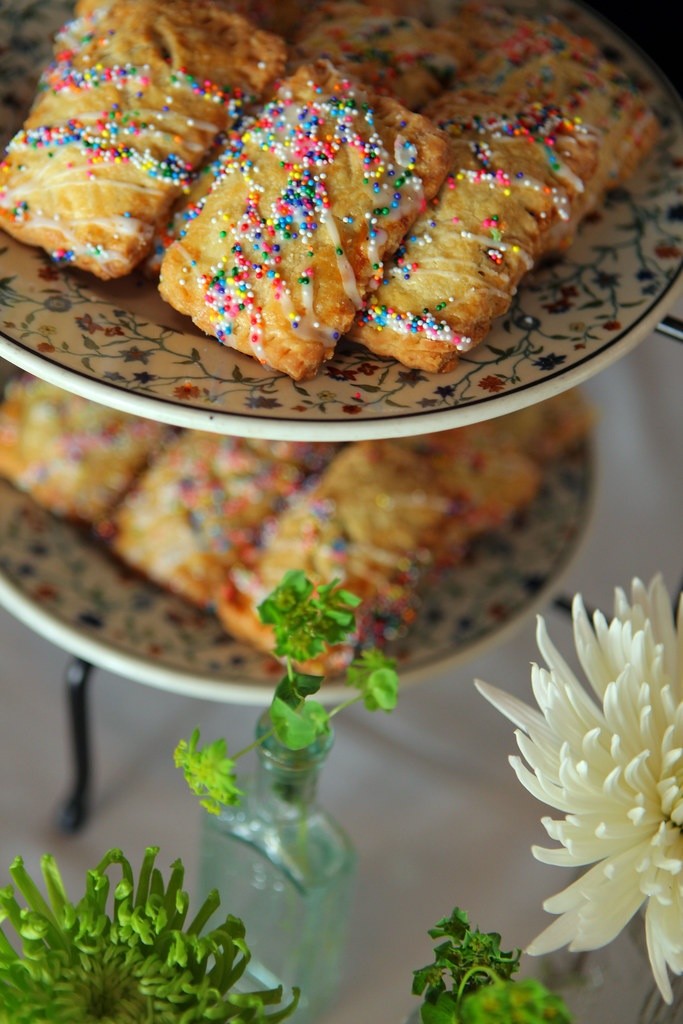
[0,356,601,707]
[0,0,683,442]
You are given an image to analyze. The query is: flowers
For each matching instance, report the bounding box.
[169,565,403,823]
[473,572,682,1005]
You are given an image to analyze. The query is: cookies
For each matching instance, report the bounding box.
[0,0,655,665]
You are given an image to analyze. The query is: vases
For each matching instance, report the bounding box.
[184,709,356,1023]
